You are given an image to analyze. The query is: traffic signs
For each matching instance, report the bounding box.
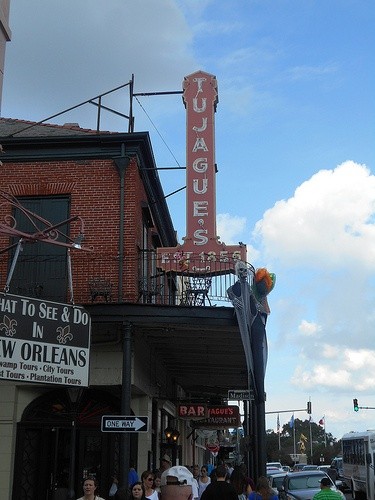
[100,415,150,432]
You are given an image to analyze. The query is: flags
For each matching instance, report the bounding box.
[289,415,293,431]
[318,417,324,424]
[276,417,280,431]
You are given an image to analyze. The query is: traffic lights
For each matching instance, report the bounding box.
[353,399,359,412]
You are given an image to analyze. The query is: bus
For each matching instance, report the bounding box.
[341,429,375,500]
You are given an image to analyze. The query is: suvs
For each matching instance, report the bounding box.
[330,457,346,488]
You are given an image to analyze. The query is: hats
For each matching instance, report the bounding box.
[160,466,193,486]
[318,477,331,485]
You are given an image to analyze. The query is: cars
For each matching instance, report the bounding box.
[279,469,346,500]
[265,462,332,496]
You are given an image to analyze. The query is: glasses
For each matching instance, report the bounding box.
[133,488,142,491]
[146,477,153,481]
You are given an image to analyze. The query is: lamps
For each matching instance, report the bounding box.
[158,426,181,445]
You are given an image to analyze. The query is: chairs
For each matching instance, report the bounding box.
[180,275,213,307]
[89,278,113,303]
[137,276,164,304]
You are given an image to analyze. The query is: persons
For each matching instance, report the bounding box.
[73,455,278,500]
[312,478,344,500]
[327,465,338,485]
